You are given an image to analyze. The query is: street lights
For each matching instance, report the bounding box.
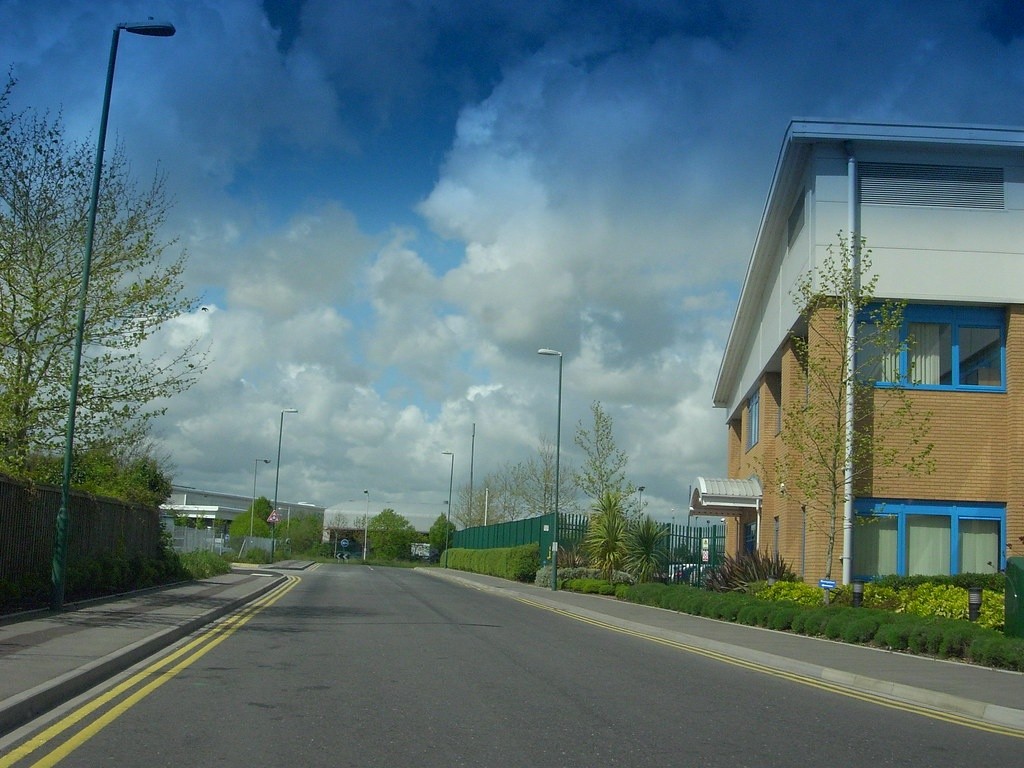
[270,408,298,564]
[46,22,178,602]
[250,458,271,536]
[537,348,562,592]
[363,489,369,560]
[443,451,454,569]
